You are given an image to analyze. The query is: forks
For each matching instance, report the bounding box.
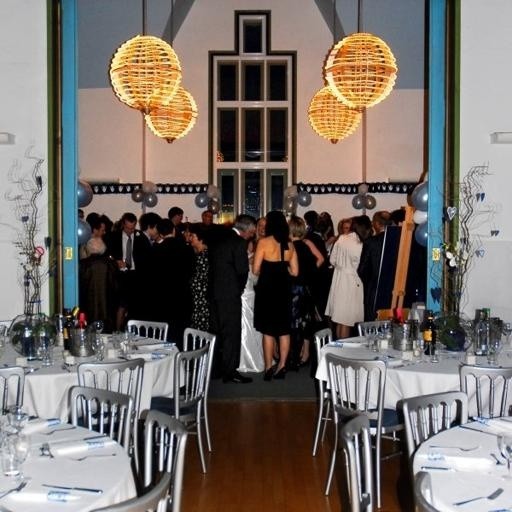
[454,487,504,506]
[429,444,481,453]
[490,453,506,466]
[1,481,28,501]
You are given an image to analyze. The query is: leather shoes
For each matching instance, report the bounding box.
[220,372,252,384]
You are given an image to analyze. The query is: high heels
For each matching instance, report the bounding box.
[263,366,274,382]
[273,366,287,381]
[296,355,311,368]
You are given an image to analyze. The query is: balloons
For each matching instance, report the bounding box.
[365,195,376,210]
[194,192,209,208]
[352,196,364,209]
[78,180,94,207]
[145,193,158,207]
[415,221,427,247]
[286,185,298,200]
[298,191,312,207]
[205,185,216,197]
[131,190,144,202]
[358,184,368,195]
[144,181,155,193]
[285,199,296,210]
[411,182,428,211]
[208,200,220,214]
[413,209,427,225]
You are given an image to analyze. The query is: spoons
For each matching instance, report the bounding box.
[73,451,118,464]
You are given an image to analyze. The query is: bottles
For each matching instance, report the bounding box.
[423,310,437,356]
[391,308,401,326]
[475,312,488,354]
[63,310,73,345]
[75,312,86,337]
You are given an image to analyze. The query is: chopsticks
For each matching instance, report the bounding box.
[42,482,104,495]
[44,426,77,435]
[36,433,110,442]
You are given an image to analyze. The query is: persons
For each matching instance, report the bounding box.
[78,208,406,384]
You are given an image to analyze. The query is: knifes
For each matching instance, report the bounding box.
[457,426,506,438]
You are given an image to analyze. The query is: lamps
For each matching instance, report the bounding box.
[111,0,181,112]
[324,1,397,113]
[143,86,197,144]
[308,86,360,144]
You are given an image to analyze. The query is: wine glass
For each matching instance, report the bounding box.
[381,321,391,339]
[493,339,503,364]
[7,405,31,439]
[417,340,428,364]
[93,321,103,335]
[496,432,512,473]
[365,321,375,350]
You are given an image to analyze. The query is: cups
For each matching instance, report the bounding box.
[0,325,8,352]
[429,341,441,364]
[413,340,421,364]
[93,334,107,361]
[2,452,20,476]
[40,340,56,368]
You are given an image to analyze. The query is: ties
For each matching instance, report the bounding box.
[124,232,133,270]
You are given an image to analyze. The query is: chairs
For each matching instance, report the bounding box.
[68,386,134,454]
[174,328,217,451]
[78,358,144,455]
[339,416,373,512]
[459,366,511,419]
[0,367,25,415]
[92,473,172,512]
[397,393,468,462]
[141,409,188,512]
[413,471,438,512]
[313,331,334,454]
[324,353,404,508]
[128,320,169,341]
[360,319,393,336]
[171,342,209,474]
